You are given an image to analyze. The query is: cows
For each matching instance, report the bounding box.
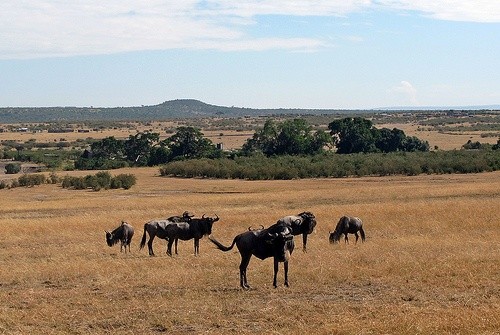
[139,210,219,258]
[104,221,134,254]
[329,215,366,245]
[209,211,317,289]
